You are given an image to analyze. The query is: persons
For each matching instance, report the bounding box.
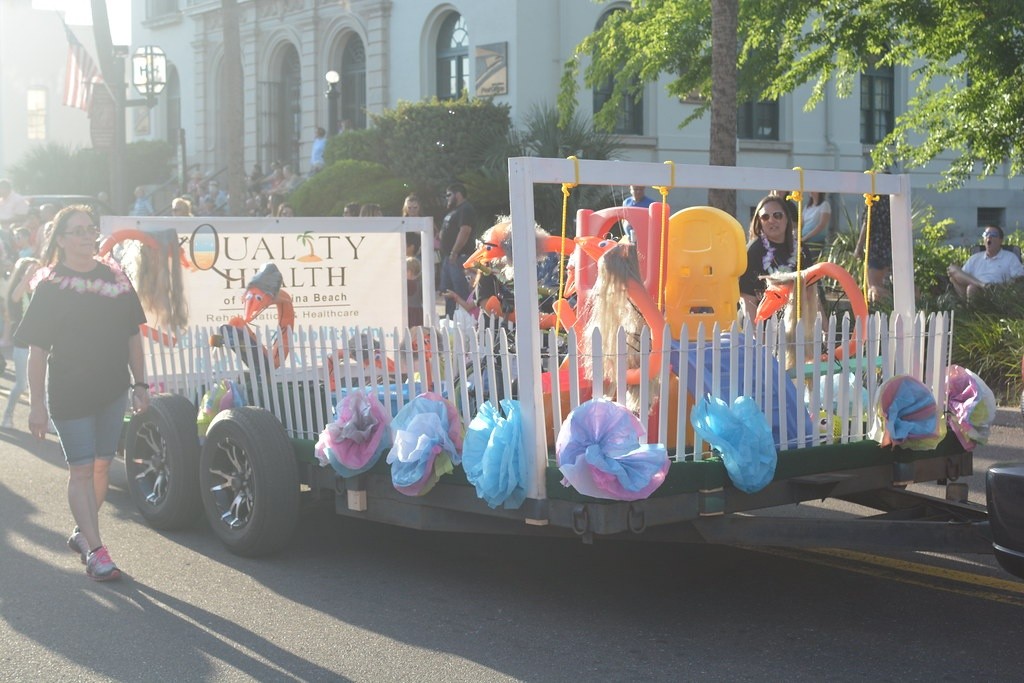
[13,207,152,581]
[945,224,1024,309]
[0,128,891,436]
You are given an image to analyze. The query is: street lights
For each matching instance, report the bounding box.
[324,70,341,136]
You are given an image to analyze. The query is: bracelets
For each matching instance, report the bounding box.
[130,382,149,389]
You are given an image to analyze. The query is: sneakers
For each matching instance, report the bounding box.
[86,543,121,581]
[65,526,88,564]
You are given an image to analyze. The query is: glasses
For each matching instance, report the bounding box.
[759,212,784,221]
[57,225,98,236]
[982,231,1001,237]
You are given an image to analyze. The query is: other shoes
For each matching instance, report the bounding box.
[2,416,13,428]
[45,419,57,434]
[439,315,454,320]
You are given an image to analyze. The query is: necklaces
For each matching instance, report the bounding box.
[760,229,802,274]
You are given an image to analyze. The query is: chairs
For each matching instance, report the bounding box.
[970,244,1021,262]
